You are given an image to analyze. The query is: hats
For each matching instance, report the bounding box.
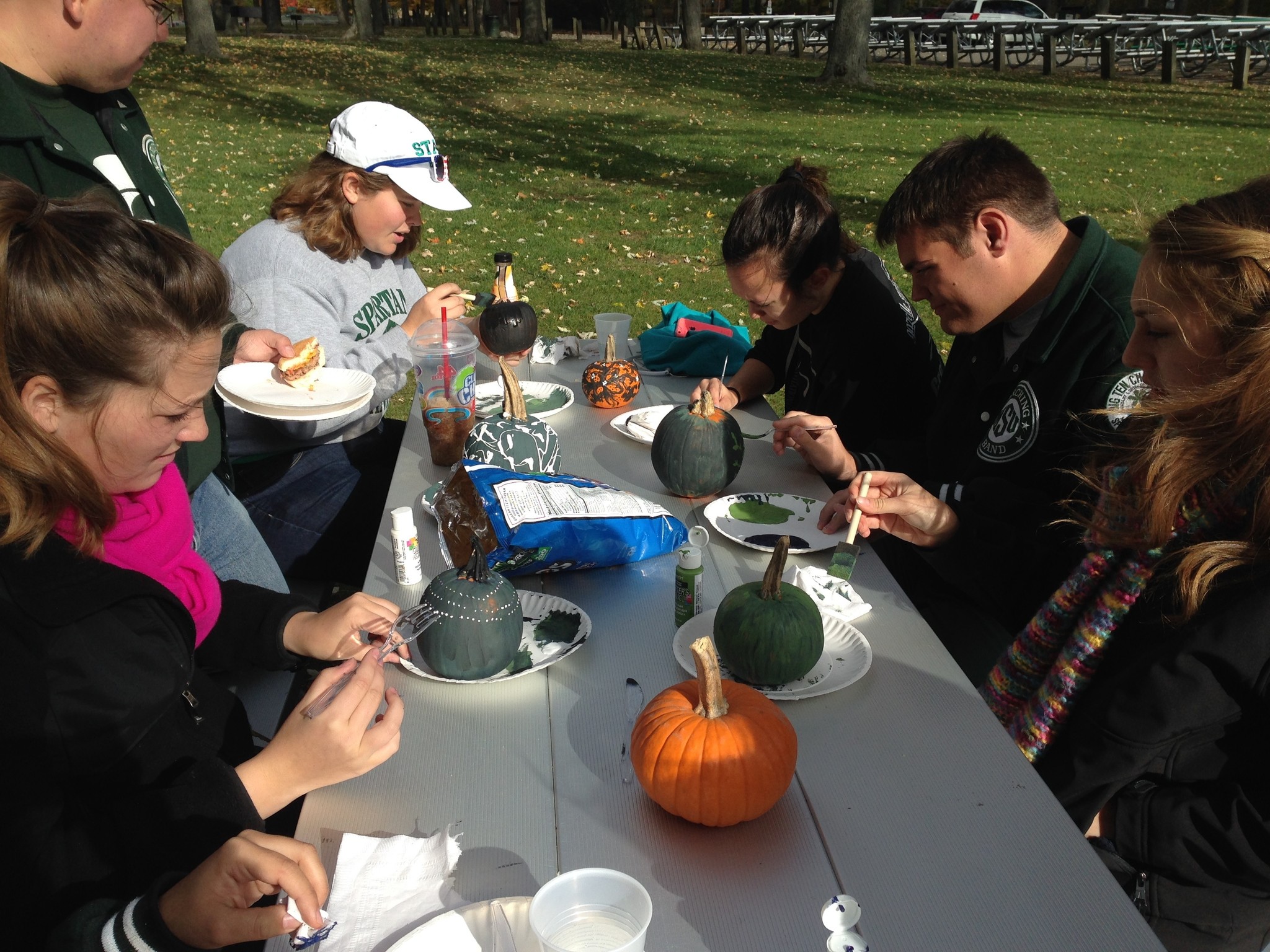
[325,101,473,211]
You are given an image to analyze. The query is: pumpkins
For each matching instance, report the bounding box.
[462,356,562,474]
[630,634,798,828]
[582,334,641,408]
[651,389,745,499]
[414,536,523,680]
[479,299,537,356]
[713,535,824,687]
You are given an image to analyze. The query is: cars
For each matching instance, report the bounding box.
[941,0,1066,47]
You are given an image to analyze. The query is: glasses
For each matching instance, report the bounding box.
[153,0,176,25]
[365,154,446,183]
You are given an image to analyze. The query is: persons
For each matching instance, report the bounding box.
[773,126,1142,687]
[691,156,945,499]
[834,175,1270,952]
[218,101,530,587]
[0,0,295,747]
[54,829,329,952]
[0,181,410,952]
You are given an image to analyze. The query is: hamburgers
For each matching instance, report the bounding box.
[278,337,326,388]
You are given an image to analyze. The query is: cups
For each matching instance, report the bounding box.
[593,313,632,360]
[408,318,479,466]
[528,867,652,952]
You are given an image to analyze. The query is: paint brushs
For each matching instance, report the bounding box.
[718,356,729,402]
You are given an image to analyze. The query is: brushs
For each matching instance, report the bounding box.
[828,472,872,579]
[427,287,497,307]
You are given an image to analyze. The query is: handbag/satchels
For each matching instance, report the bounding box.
[640,302,753,376]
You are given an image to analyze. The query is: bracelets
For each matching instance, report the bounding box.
[727,387,741,408]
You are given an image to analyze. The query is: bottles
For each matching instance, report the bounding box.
[492,252,520,302]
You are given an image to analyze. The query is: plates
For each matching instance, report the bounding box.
[703,492,860,554]
[672,607,873,700]
[400,589,592,684]
[610,404,682,446]
[474,381,574,419]
[213,362,376,422]
[383,894,555,952]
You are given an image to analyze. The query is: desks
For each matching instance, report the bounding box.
[263,335,1167,952]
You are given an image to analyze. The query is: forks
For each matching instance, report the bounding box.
[743,425,837,439]
[306,603,441,718]
